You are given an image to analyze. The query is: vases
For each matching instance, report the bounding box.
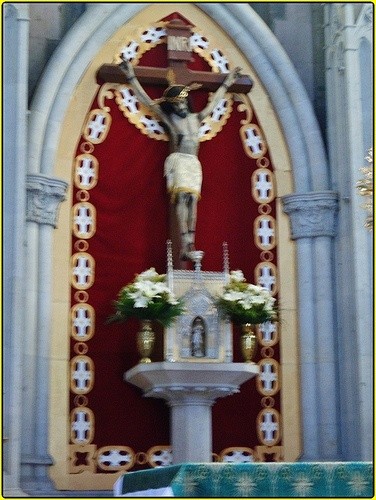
[240,322,258,364]
[136,320,158,364]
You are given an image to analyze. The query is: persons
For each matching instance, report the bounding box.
[192,320,204,353]
[118,58,242,261]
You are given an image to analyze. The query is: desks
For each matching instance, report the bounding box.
[114,460,373,496]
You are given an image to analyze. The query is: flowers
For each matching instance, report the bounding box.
[103,267,187,327]
[209,269,287,325]
